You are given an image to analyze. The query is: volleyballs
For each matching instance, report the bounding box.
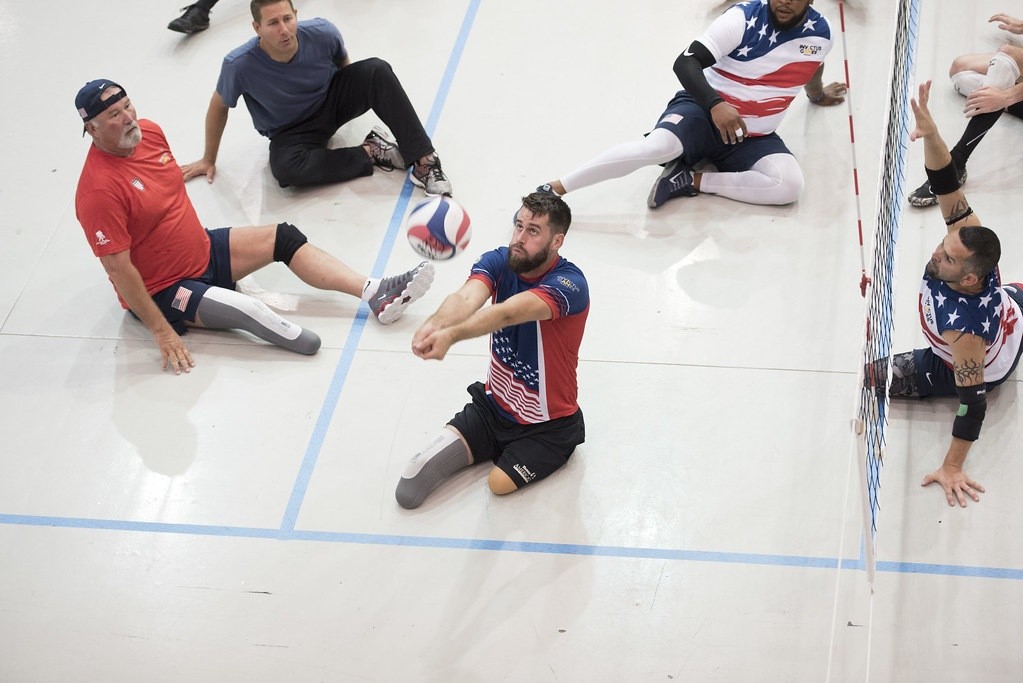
[406,196,470,262]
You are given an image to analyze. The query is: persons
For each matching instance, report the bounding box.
[513,0,847,223]
[167,0,218,34]
[176,0,452,197]
[76,79,435,374]
[906,12,1023,206]
[393,191,589,509]
[863,78,1023,507]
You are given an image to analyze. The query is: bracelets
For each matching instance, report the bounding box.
[921,158,960,194]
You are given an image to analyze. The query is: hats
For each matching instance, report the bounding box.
[75,80,127,137]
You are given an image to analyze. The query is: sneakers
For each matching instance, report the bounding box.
[646,155,699,208]
[909,169,968,208]
[410,153,452,197]
[369,260,435,325]
[362,125,407,171]
[167,3,210,33]
[512,183,561,223]
